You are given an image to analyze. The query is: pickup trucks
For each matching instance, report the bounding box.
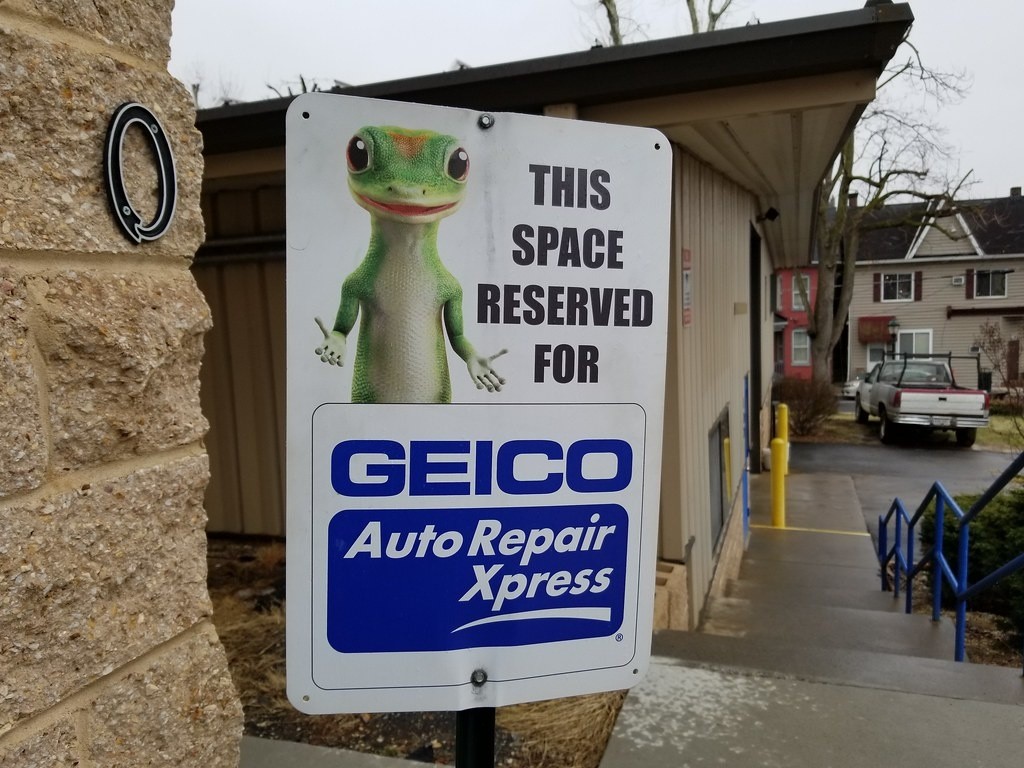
[854,350,990,447]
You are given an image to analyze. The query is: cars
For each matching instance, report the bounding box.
[841,368,938,401]
